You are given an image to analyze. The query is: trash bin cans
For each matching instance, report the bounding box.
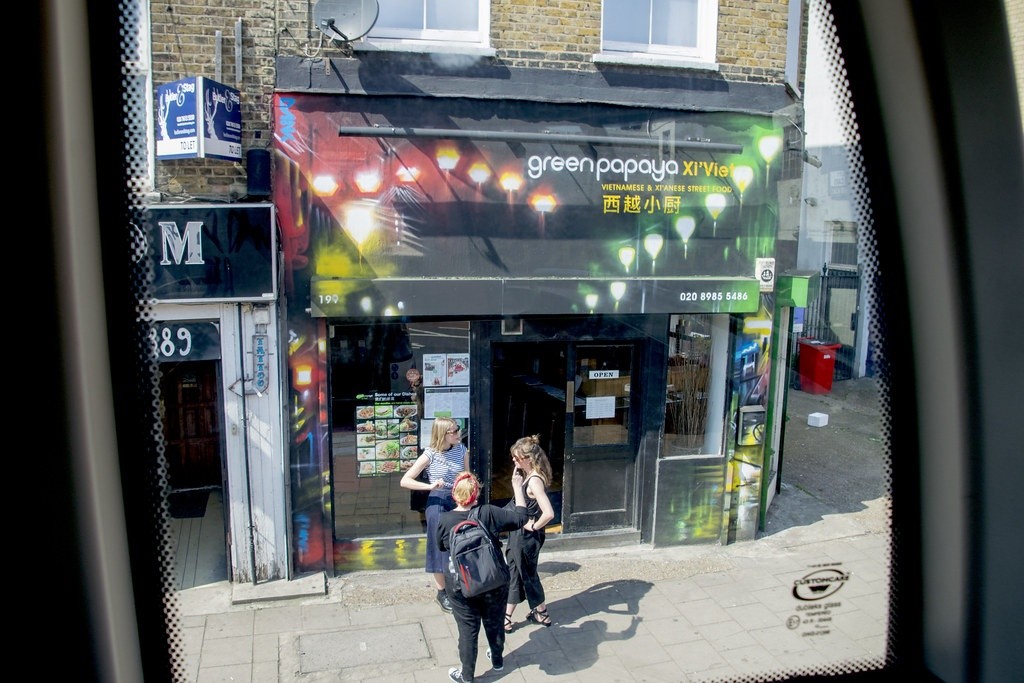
[796,337,841,395]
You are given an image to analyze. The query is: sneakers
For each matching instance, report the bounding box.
[486,647,504,671]
[448,667,466,683]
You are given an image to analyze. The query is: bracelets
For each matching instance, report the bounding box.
[531,524,536,531]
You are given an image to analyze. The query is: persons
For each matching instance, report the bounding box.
[437,465,529,683]
[504,433,554,634]
[399,417,477,614]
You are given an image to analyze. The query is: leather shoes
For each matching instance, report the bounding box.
[435,592,454,613]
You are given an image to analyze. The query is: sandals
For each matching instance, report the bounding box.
[526,609,551,627]
[503,614,513,634]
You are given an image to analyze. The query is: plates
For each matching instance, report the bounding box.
[400,437,417,445]
[399,421,417,431]
[402,446,417,458]
[394,405,418,418]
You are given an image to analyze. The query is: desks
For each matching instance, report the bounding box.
[493,368,708,465]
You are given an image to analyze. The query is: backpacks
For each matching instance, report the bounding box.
[449,505,510,597]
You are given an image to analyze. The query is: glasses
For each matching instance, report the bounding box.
[513,454,531,462]
[446,425,461,434]
